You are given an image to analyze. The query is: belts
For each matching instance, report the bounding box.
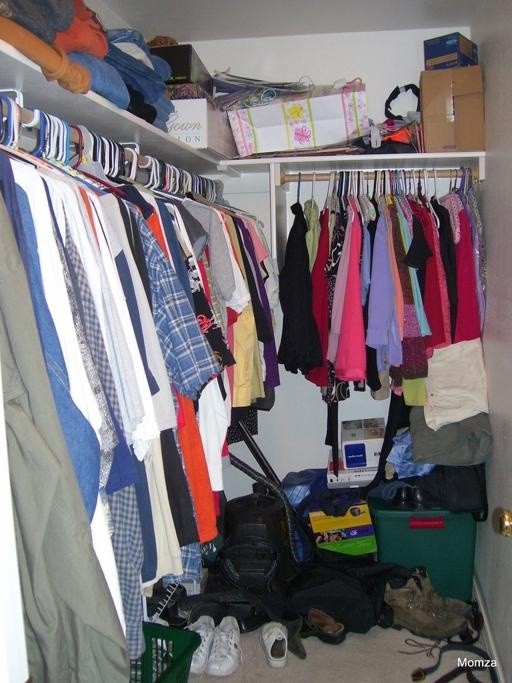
[412,642,499,683]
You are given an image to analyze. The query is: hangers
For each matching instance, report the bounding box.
[0,87,267,234]
[288,169,483,240]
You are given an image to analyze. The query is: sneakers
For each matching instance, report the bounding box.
[383,578,465,640]
[185,615,214,673]
[412,567,471,616]
[205,616,240,675]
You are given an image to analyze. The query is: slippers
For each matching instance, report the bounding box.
[300,608,346,645]
[262,623,289,666]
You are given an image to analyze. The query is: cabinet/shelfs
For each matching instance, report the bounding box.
[200,149,486,488]
[2,40,241,677]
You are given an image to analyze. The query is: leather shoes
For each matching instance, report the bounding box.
[411,487,432,511]
[384,486,410,509]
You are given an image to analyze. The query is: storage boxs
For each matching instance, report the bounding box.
[308,506,377,541]
[423,32,479,70]
[373,500,477,608]
[152,41,215,95]
[418,71,486,150]
[129,619,202,677]
[164,98,236,160]
[323,460,382,487]
[340,418,387,466]
[317,538,377,558]
